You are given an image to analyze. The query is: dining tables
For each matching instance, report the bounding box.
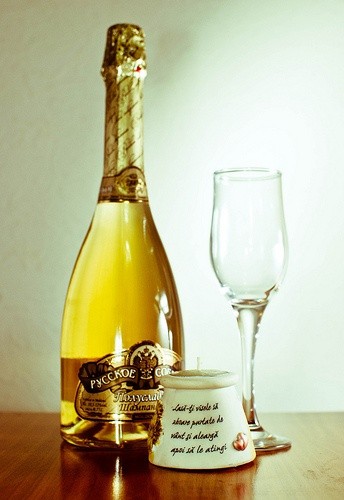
[1,409,343,499]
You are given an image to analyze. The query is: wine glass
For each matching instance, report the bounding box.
[210,168,293,451]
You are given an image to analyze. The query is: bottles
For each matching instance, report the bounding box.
[60,23,185,452]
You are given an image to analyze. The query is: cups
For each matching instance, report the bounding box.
[147,369,256,469]
